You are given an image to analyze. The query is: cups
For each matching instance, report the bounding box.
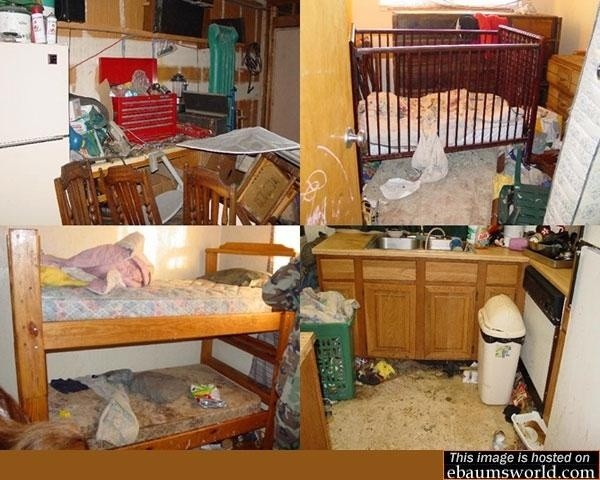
[146,83,161,95]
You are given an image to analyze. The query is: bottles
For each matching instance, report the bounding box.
[31,2,57,44]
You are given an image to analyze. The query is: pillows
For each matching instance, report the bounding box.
[198,269,273,289]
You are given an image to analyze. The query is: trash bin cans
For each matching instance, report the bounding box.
[477,294,526,406]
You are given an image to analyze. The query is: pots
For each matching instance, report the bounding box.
[1,3,31,43]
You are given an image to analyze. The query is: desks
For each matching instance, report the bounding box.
[390,9,562,105]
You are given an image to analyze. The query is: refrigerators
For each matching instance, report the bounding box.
[1,41,70,225]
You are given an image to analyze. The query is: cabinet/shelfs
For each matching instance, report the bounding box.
[547,52,588,138]
[316,255,527,360]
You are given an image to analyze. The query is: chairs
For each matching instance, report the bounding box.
[53,155,240,225]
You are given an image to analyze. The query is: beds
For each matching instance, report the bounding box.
[6,229,298,448]
[349,24,547,195]
